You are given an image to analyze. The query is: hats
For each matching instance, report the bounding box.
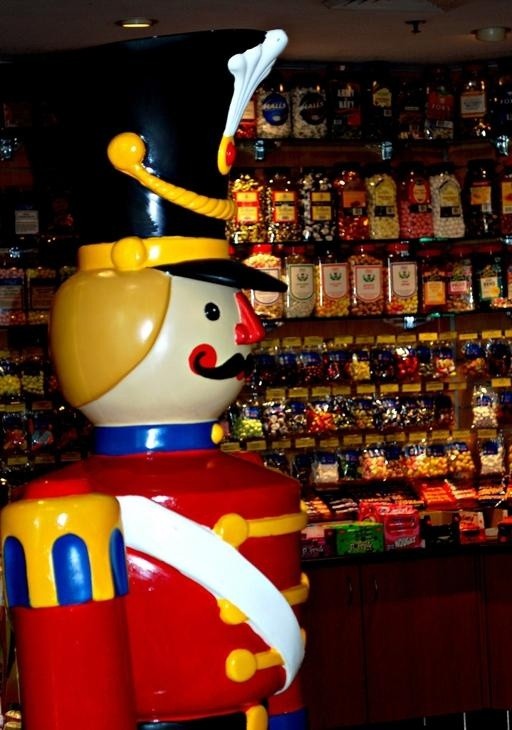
[74,29,290,297]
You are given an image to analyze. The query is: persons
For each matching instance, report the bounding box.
[0,29,308,728]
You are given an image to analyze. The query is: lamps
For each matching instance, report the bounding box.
[114,18,156,28]
[470,27,509,42]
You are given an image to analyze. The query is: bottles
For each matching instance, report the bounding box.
[399,159,433,239]
[327,65,362,144]
[428,162,465,239]
[242,243,283,319]
[282,245,314,319]
[473,242,506,311]
[292,68,327,142]
[265,168,300,242]
[461,159,498,238]
[347,242,384,318]
[395,71,423,141]
[444,246,473,312]
[384,242,418,316]
[415,249,445,313]
[365,163,399,240]
[334,162,369,240]
[298,164,335,242]
[257,76,292,140]
[498,156,512,236]
[458,63,486,138]
[313,245,350,318]
[227,169,265,242]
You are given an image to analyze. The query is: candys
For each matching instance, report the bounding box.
[0,266,77,327]
[229,387,512,441]
[263,439,512,486]
[0,367,58,399]
[247,335,512,389]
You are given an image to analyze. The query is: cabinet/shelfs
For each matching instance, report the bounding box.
[212,76,510,729]
[0,152,93,514]
